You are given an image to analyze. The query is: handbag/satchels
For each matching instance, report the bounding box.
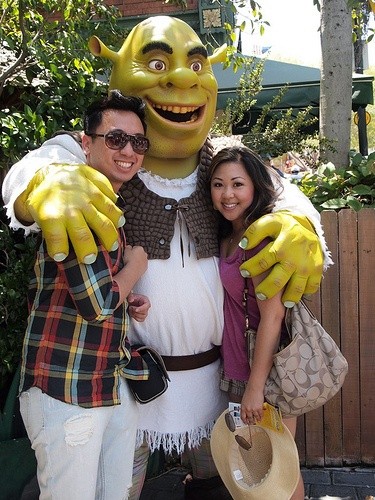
[241,251,349,418]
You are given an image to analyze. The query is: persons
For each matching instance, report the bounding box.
[208,146,305,500]
[2,15,334,500]
[16,88,152,500]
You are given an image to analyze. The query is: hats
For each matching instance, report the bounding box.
[210,408,300,500]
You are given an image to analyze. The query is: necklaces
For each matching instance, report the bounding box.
[229,239,238,246]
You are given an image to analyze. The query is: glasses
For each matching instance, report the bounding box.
[225,410,252,451]
[87,129,151,155]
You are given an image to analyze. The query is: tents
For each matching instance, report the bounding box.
[211,52,375,156]
